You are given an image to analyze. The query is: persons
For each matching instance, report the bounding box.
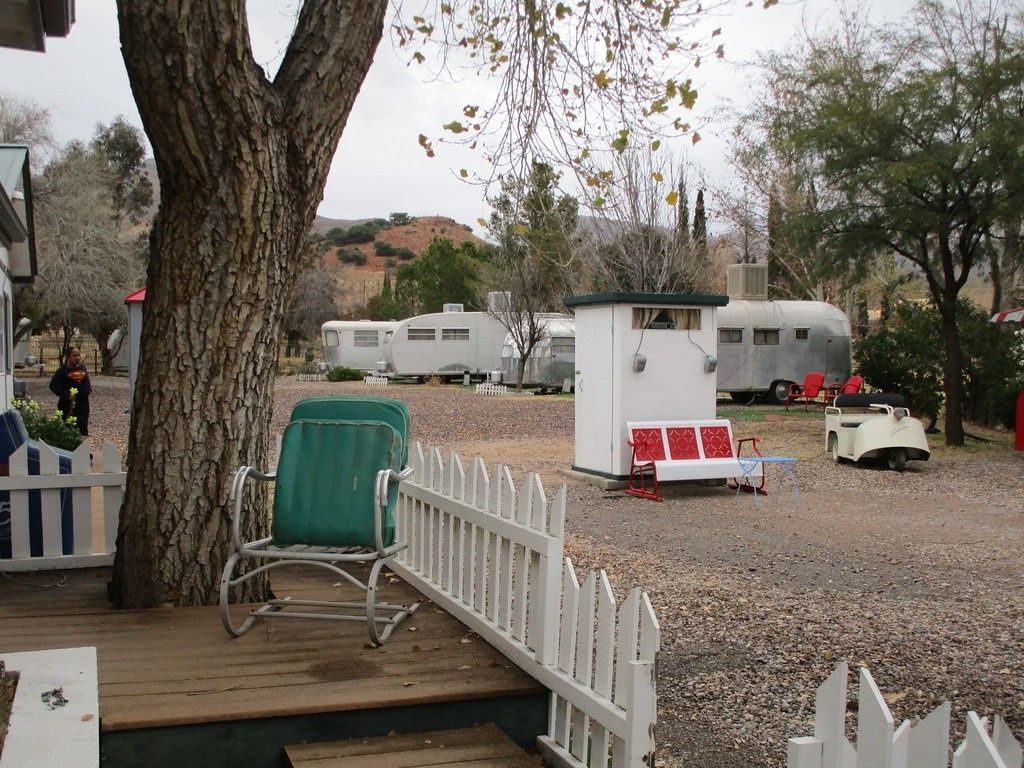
[49,346,92,435]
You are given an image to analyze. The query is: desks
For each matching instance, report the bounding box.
[735,457,802,506]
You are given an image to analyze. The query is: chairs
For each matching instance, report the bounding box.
[219,396,424,645]
[786,374,864,413]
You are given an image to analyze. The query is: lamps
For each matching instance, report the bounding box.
[688,310,717,375]
[632,309,647,374]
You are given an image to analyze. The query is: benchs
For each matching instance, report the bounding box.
[826,393,911,424]
[622,419,769,502]
